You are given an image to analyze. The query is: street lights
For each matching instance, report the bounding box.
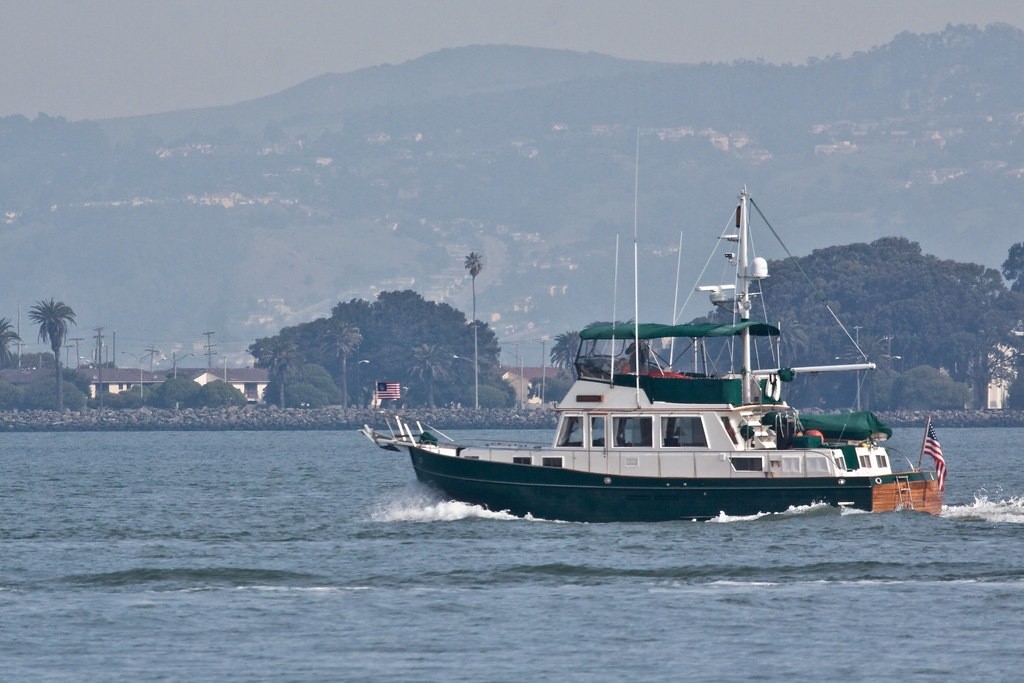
[342,358,370,412]
[121,351,162,397]
[158,350,198,379]
[451,353,479,410]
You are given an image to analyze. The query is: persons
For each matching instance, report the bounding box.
[620,358,631,374]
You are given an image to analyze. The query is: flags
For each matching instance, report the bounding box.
[376,381,401,399]
[924,422,946,492]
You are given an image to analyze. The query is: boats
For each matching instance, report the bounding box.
[354,185,952,522]
